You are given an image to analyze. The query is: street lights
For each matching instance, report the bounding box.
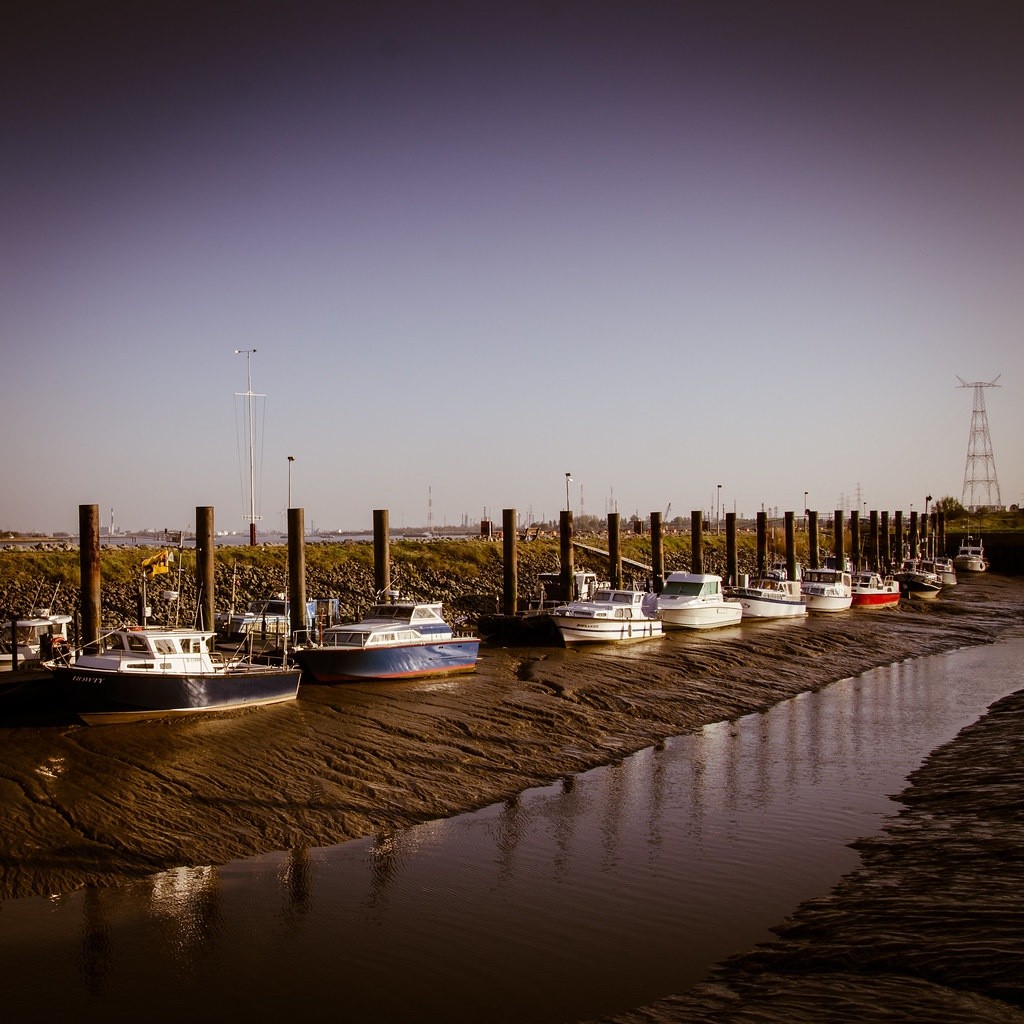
[288,456,295,509]
[717,485,722,534]
[805,491,808,513]
[565,473,570,510]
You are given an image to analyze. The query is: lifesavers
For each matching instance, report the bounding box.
[51,637,69,659]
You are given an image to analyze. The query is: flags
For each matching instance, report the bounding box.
[141,551,168,574]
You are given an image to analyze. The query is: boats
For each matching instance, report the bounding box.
[40,531,304,726]
[919,528,957,588]
[468,573,611,647]
[723,566,807,616]
[820,547,855,573]
[850,572,902,609]
[894,560,944,599]
[288,576,483,682]
[766,557,804,580]
[657,570,743,630]
[212,557,341,653]
[801,569,853,612]
[953,516,990,572]
[0,576,83,726]
[547,578,666,649]
